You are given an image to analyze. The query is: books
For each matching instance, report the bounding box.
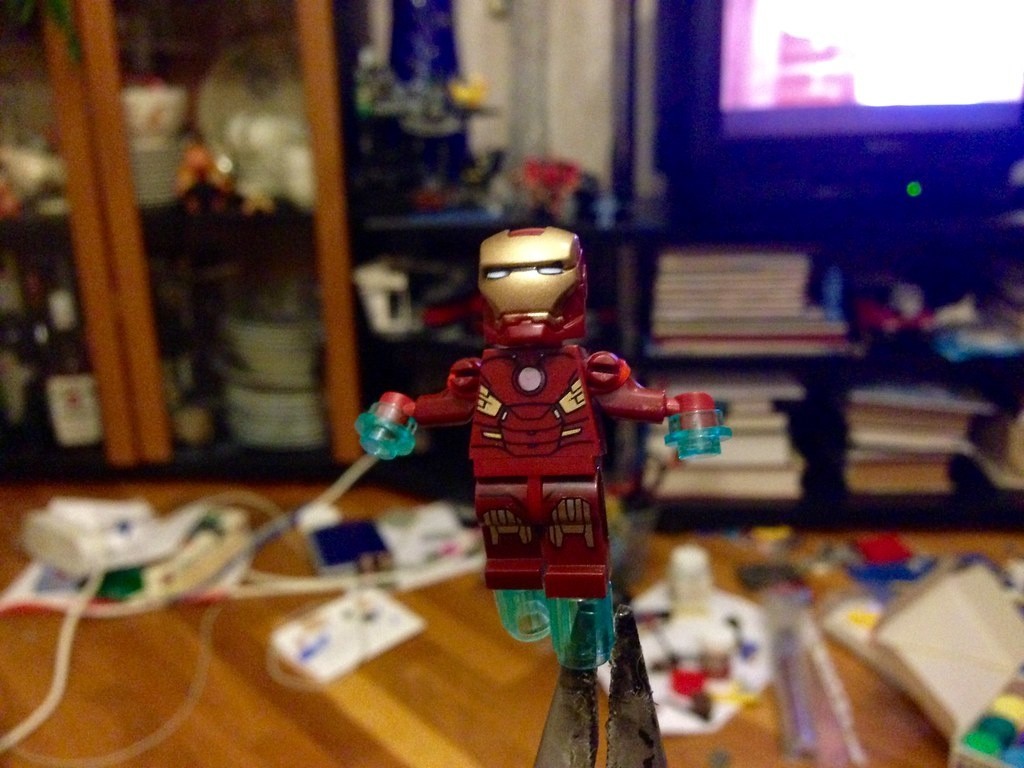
[845,387,993,496]
[645,253,850,355]
[643,374,805,500]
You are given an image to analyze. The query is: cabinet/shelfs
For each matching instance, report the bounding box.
[332,0,636,502]
[0,0,363,487]
[624,190,1024,538]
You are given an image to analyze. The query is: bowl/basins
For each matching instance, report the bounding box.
[124,84,190,146]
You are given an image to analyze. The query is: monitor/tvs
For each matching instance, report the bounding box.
[652,0,1024,216]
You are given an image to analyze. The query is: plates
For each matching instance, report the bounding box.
[129,148,184,209]
[207,311,328,452]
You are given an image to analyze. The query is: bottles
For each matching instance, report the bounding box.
[0,240,199,469]
[820,262,848,324]
